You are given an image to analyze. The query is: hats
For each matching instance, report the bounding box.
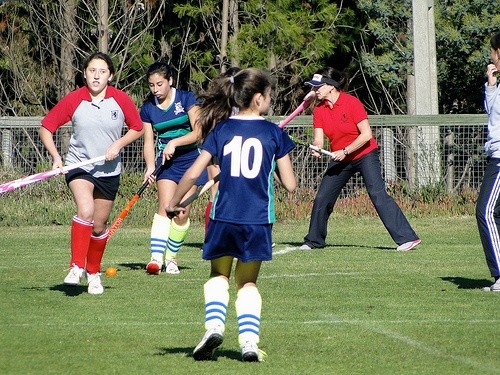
[304,74,340,90]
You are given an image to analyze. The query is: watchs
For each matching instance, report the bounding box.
[343,147,349,156]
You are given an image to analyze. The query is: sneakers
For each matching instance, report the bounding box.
[193,328,223,361]
[300,245,311,250]
[490,278,500,291]
[397,240,421,251]
[241,341,268,362]
[64,263,84,286]
[87,272,104,294]
[146,258,180,275]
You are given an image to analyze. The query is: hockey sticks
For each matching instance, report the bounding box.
[105,161,164,243]
[286,132,334,160]
[0,154,105,194]
[167,90,317,219]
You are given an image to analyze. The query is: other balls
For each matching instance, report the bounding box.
[105,268,116,278]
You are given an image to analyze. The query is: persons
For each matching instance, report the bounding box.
[38,51,146,295]
[298,66,422,251]
[475,33,500,292]
[165,67,297,362]
[139,62,209,275]
